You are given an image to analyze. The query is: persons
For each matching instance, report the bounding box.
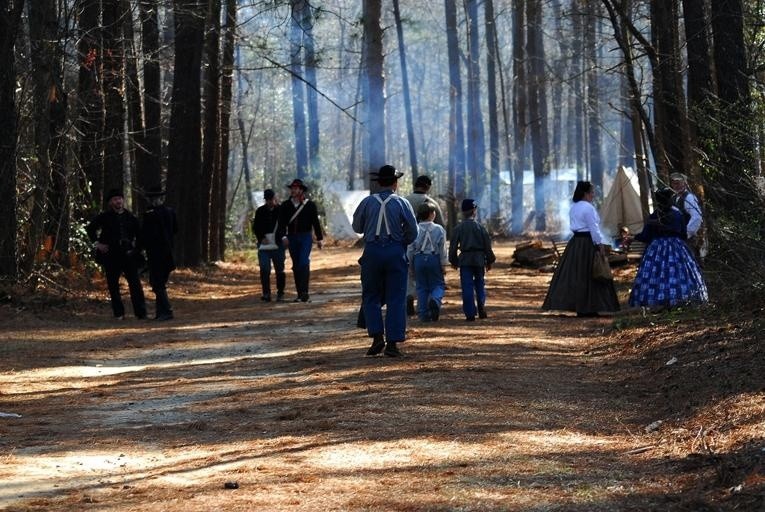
[133,181,190,320]
[252,188,287,300]
[281,179,322,301]
[351,165,497,357]
[629,186,712,309]
[671,171,704,243]
[542,181,621,318]
[85,188,147,320]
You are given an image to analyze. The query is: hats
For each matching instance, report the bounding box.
[263,188,276,200]
[142,182,167,197]
[104,187,125,203]
[460,198,479,211]
[286,178,308,193]
[368,165,405,182]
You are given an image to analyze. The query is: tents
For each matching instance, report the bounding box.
[237,193,265,232]
[599,165,654,235]
[328,188,370,238]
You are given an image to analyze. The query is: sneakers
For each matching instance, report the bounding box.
[428,297,442,322]
[261,290,311,303]
[406,294,417,318]
[463,309,489,320]
[384,340,405,358]
[113,310,175,322]
[366,335,386,355]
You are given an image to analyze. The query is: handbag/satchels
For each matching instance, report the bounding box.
[258,232,280,251]
